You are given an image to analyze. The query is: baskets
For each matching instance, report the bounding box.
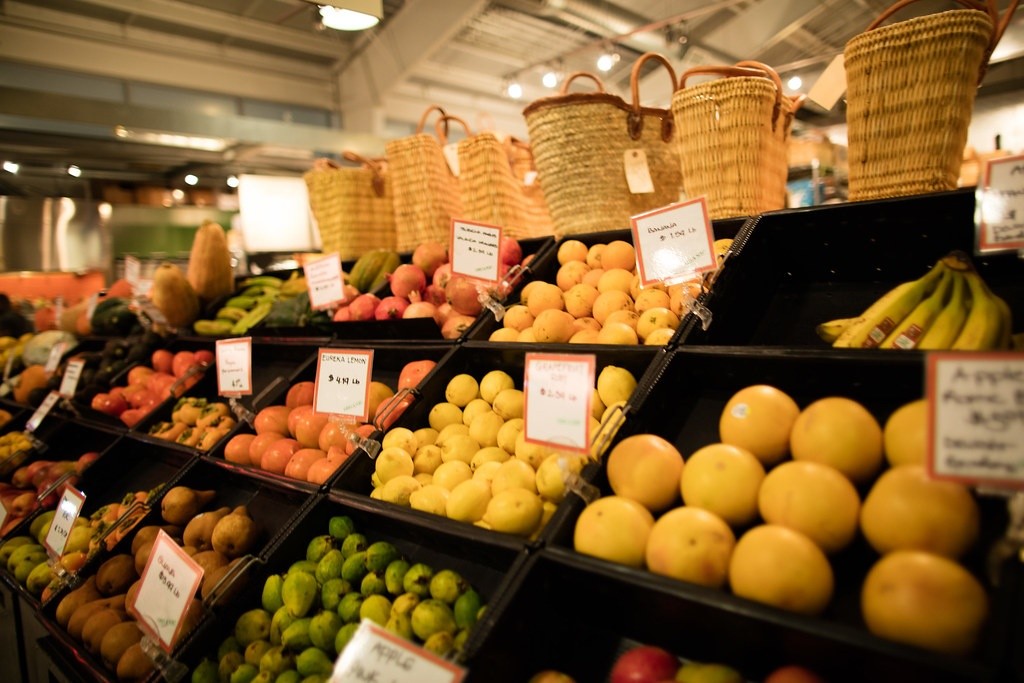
[845,0,1018,204]
[671,59,807,220]
[459,132,557,237]
[384,104,471,252]
[521,53,682,237]
[305,153,398,261]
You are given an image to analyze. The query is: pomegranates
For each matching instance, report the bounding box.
[333,239,535,340]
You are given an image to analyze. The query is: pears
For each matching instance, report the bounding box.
[56,487,256,683]
[0,510,87,594]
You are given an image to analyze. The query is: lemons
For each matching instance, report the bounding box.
[369,363,636,541]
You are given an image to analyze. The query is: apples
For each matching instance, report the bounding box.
[525,645,818,683]
[0,451,99,536]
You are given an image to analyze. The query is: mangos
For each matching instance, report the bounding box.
[92,349,211,426]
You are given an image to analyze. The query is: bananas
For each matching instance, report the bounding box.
[815,254,1011,354]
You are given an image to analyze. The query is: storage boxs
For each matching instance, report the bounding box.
[0,184,1024,683]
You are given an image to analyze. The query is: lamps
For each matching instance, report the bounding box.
[595,45,622,73]
[541,58,568,89]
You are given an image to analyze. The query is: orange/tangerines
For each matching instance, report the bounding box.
[573,385,986,657]
[486,240,733,345]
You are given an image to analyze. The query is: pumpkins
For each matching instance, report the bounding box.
[1,219,235,400]
[266,247,401,324]
